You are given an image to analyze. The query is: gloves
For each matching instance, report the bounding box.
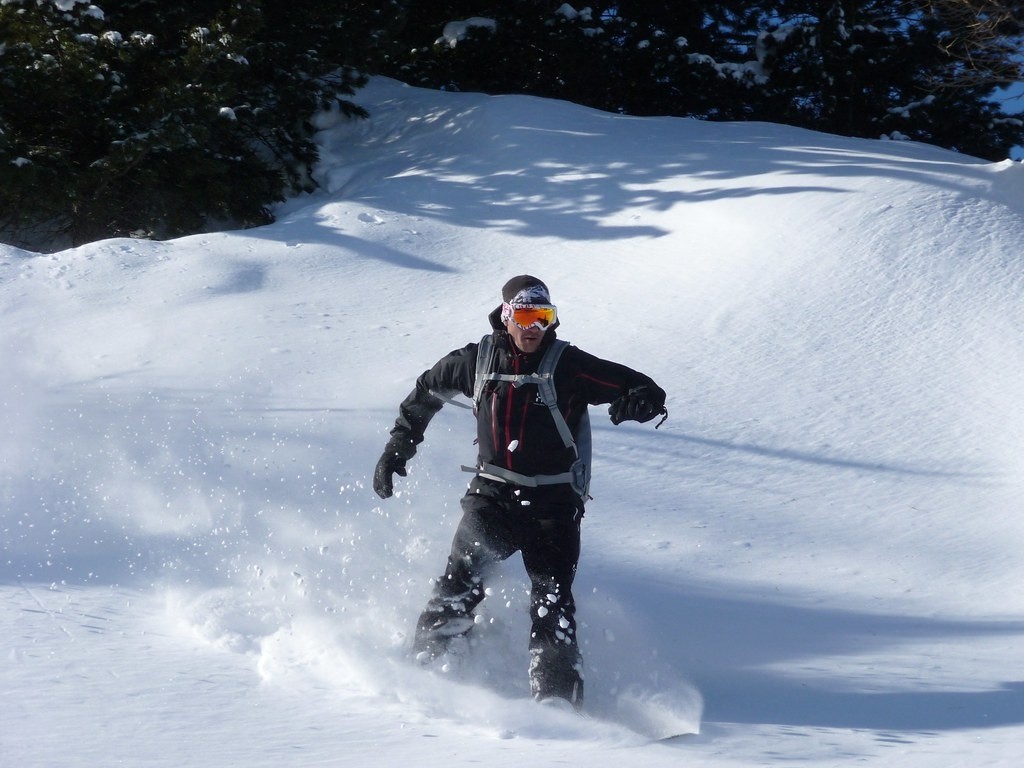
[608,395,659,426]
[372,443,417,499]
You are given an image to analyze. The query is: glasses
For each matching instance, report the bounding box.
[511,304,557,331]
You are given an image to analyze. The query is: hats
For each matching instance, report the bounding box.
[502,274,550,320]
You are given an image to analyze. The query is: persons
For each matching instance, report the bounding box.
[373,274,667,716]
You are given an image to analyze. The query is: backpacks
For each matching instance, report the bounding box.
[472,334,593,504]
